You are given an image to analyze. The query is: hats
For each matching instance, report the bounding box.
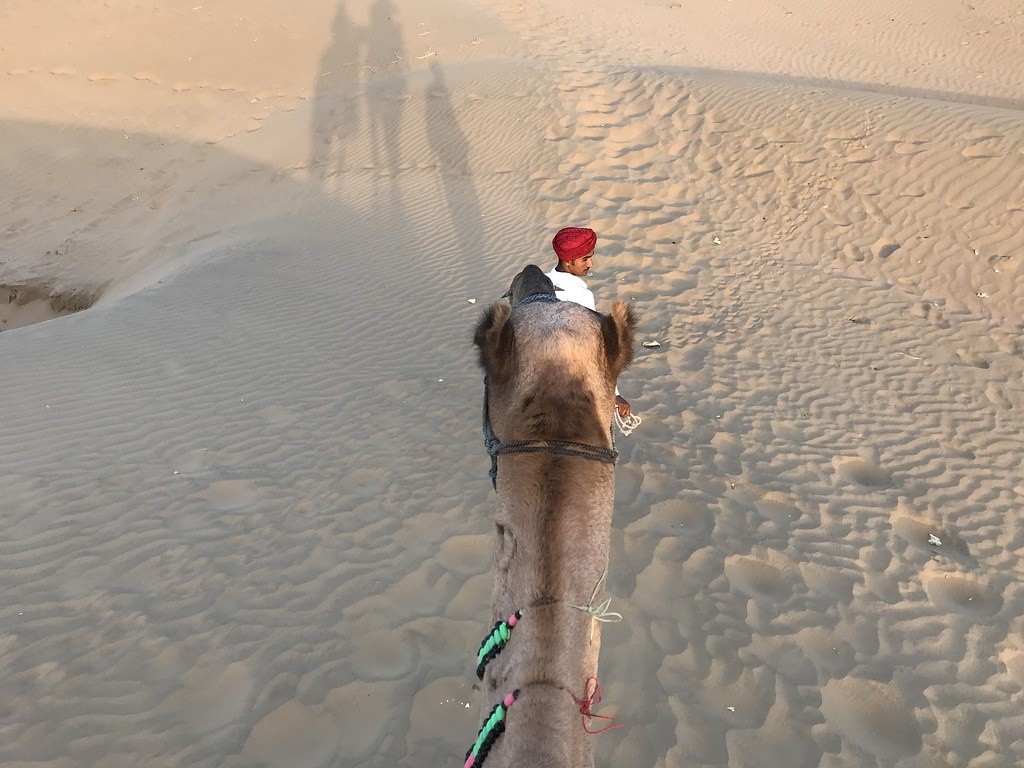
[553,227,597,261]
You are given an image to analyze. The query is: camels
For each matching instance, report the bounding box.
[469,265,637,768]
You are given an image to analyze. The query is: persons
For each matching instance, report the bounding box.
[544,227,631,419]
